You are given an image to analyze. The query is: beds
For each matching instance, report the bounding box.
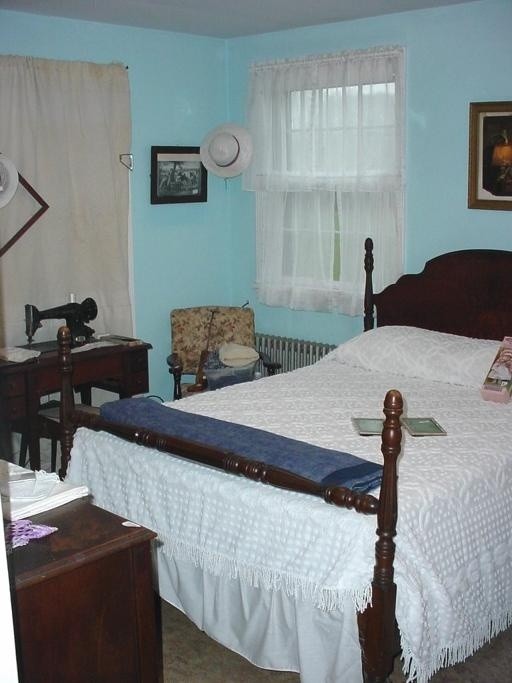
[59,250,511,683]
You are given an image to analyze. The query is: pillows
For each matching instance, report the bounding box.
[326,324,502,388]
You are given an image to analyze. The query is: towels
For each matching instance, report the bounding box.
[2,457,88,521]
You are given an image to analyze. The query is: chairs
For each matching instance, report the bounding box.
[167,306,281,400]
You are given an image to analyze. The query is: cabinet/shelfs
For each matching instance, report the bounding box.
[0,350,148,423]
[6,497,161,682]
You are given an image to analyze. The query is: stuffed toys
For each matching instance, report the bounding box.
[487,342,512,386]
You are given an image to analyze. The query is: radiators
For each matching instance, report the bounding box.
[254,332,337,379]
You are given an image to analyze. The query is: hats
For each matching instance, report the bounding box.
[0,153,18,208]
[200,123,252,177]
[219,343,258,366]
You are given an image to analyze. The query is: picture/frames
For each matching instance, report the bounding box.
[469,101,512,210]
[151,144,207,204]
[351,416,447,436]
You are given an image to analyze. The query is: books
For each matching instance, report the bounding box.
[1,454,94,523]
